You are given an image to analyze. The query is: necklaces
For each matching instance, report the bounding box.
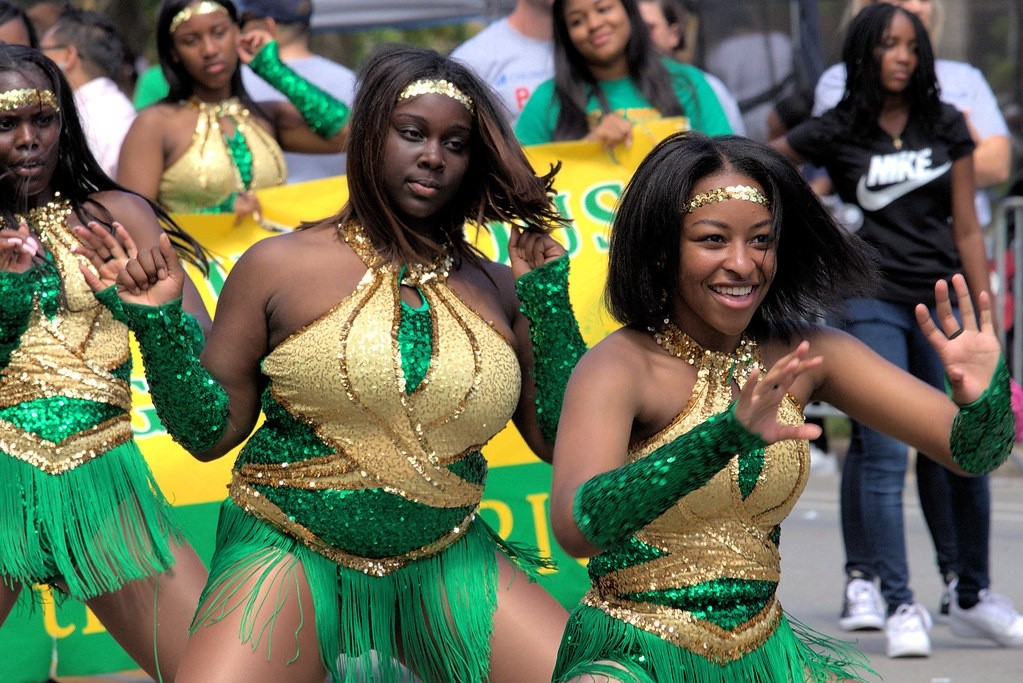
[879,124,908,150]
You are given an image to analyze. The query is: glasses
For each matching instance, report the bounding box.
[238,10,260,30]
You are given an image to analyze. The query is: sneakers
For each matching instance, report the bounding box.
[938,580,953,622]
[946,578,1023,648]
[838,574,887,630]
[883,602,930,658]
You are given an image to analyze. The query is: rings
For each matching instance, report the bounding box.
[103,255,114,263]
[774,384,779,390]
[949,330,966,340]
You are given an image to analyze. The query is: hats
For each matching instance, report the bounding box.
[242,0,312,22]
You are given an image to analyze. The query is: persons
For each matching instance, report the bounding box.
[811,0,1012,630]
[704,7,797,146]
[112,43,591,682]
[0,39,215,683]
[768,84,833,201]
[551,128,1018,682]
[769,0,1023,658]
[0,0,747,225]
